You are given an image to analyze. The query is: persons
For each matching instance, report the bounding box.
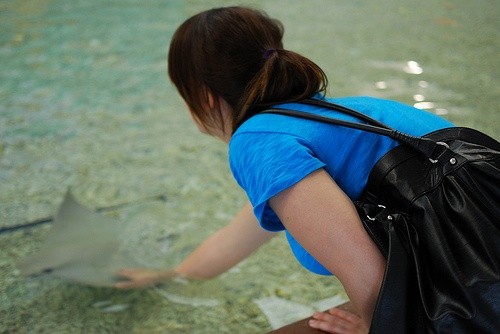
[114,6,459,334]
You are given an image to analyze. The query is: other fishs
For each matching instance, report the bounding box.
[18,187,174,290]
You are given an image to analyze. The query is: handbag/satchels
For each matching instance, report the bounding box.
[359,127,500,334]
